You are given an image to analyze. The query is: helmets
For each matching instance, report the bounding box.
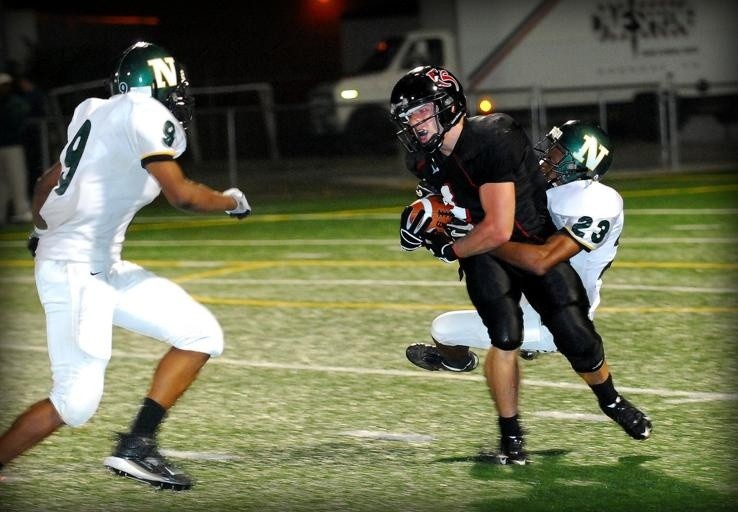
[532,118,616,188]
[110,40,196,131]
[386,66,467,155]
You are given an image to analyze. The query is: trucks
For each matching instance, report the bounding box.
[308,0,738,156]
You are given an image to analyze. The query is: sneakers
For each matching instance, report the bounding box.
[405,343,479,373]
[222,187,252,220]
[499,433,527,466]
[598,391,653,441]
[518,349,538,360]
[103,431,193,491]
[27,225,48,257]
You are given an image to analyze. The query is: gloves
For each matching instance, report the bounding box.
[399,181,475,264]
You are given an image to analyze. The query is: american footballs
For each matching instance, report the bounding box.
[409,195,454,233]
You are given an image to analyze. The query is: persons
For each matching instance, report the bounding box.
[387,65,652,465]
[404,118,623,374]
[0,72,34,226]
[0,38,251,494]
